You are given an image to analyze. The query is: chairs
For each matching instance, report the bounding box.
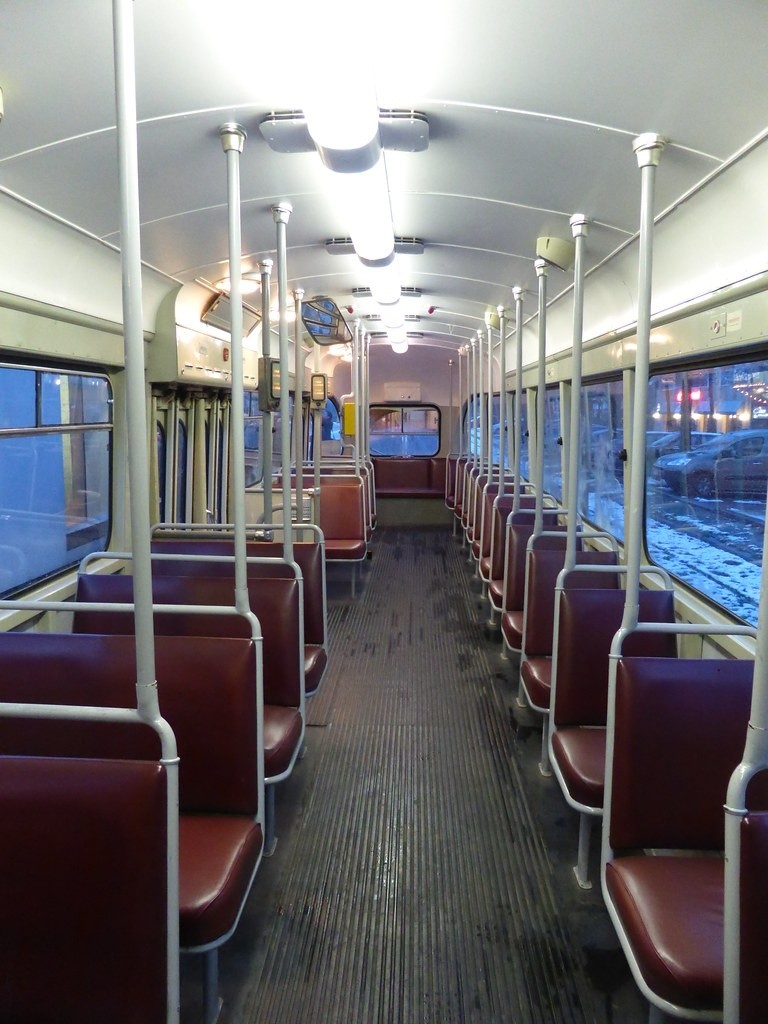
[444,451,768,1024]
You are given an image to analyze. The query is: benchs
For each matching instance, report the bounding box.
[0,455,447,1023]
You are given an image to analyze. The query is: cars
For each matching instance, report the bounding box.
[649,427,768,499]
[493,417,725,475]
[467,415,498,428]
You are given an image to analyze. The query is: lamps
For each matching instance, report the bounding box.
[260,73,430,352]
